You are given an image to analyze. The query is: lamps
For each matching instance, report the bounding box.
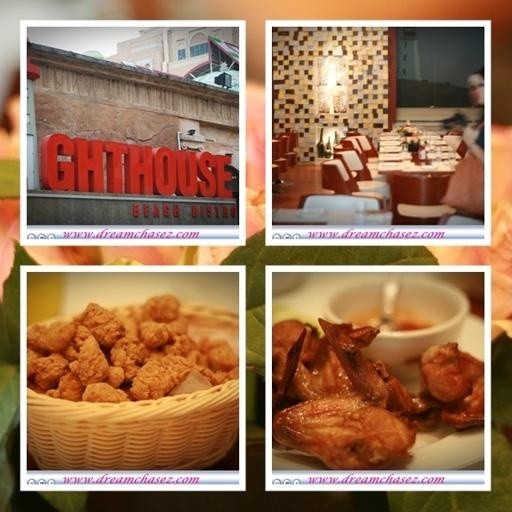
[214,72,233,90]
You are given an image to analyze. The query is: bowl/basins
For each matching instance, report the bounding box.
[321,277,470,383]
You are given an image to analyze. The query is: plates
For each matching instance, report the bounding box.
[272,285,484,470]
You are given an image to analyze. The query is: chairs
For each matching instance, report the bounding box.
[298,128,479,225]
[272,130,299,195]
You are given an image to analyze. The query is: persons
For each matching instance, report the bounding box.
[439,124,484,220]
[448,72,483,137]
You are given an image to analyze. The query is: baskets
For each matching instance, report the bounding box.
[25,299,239,471]
